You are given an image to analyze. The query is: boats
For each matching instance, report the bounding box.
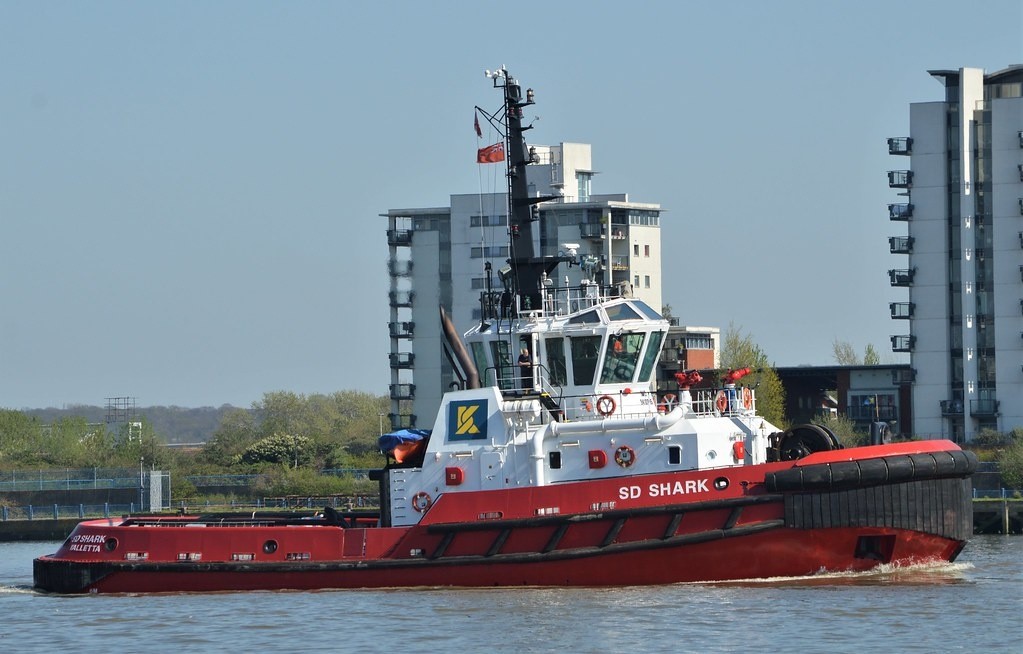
[31,64,984,599]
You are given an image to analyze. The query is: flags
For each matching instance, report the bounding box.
[474,112,482,136]
[863,397,875,406]
[478,141,504,163]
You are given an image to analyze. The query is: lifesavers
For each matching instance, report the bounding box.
[614,445,635,467]
[595,396,617,415]
[742,387,751,410]
[717,390,727,413]
[412,492,432,512]
[660,394,678,413]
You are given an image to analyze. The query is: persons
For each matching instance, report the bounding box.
[314,511,324,524]
[518,348,532,391]
